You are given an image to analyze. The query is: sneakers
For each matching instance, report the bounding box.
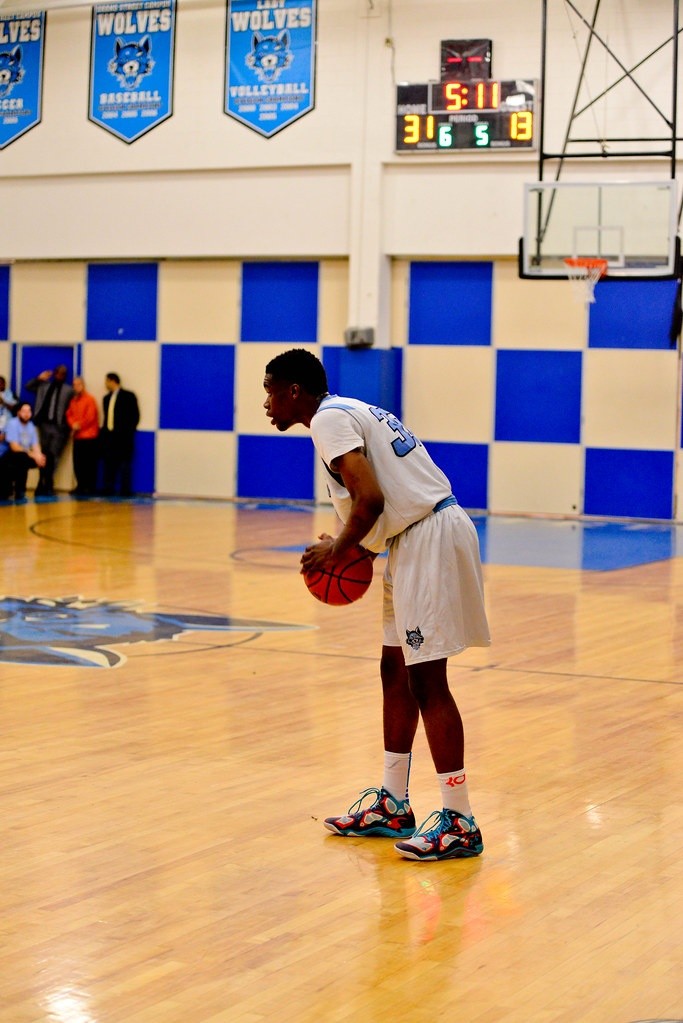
[325,788,416,838]
[394,807,482,862]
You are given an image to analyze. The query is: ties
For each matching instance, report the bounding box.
[22,425,30,451]
[108,391,116,430]
[48,387,58,420]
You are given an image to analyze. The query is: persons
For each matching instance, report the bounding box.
[0,365,140,508]
[263,350,483,861]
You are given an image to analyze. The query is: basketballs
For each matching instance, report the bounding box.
[303,544,374,606]
